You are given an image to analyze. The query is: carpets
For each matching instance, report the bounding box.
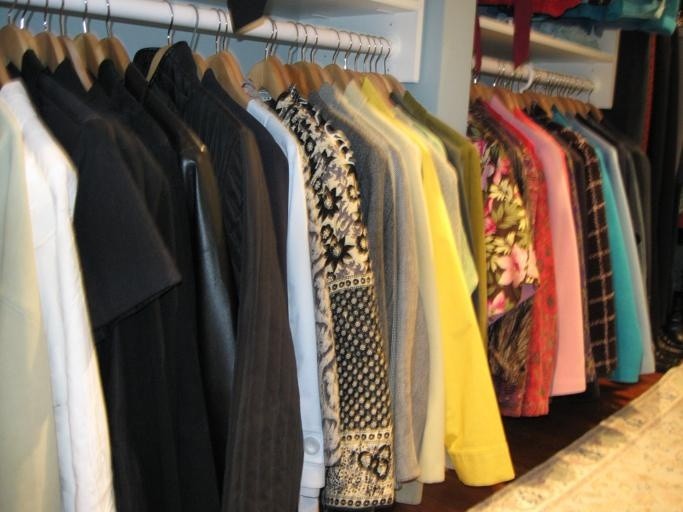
[469,363,683,504]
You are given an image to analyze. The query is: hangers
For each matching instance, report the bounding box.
[3,1,408,109]
[471,57,608,123]
